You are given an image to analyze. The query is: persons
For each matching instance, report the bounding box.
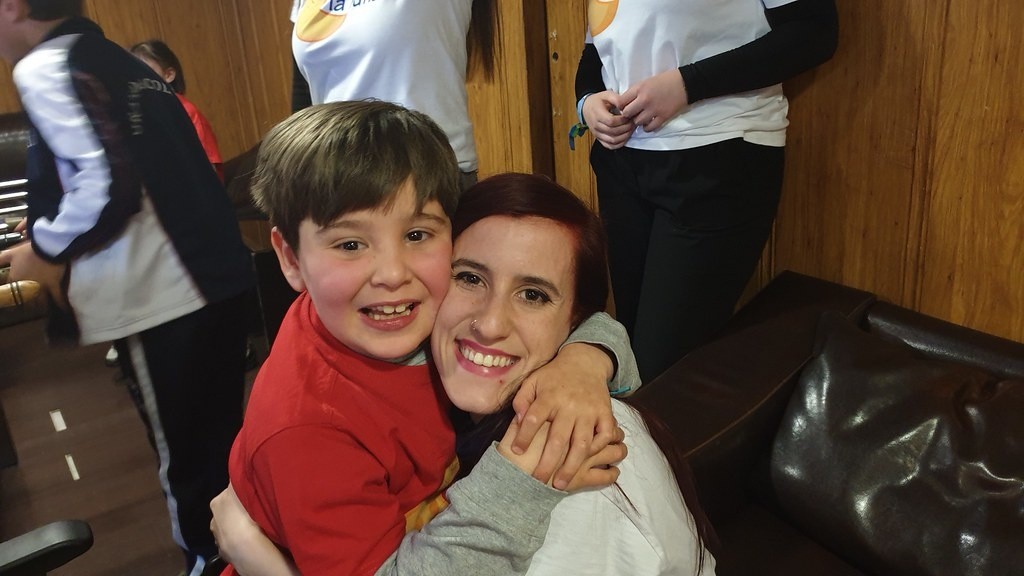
[573,0,840,387]
[208,99,725,575]
[287,0,507,193]
[0,0,239,576]
[127,37,259,379]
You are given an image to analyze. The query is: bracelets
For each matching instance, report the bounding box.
[567,93,594,152]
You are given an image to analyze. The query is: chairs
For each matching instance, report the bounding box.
[621,271,1024,576]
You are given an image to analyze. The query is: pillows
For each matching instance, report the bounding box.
[770,308,1024,576]
